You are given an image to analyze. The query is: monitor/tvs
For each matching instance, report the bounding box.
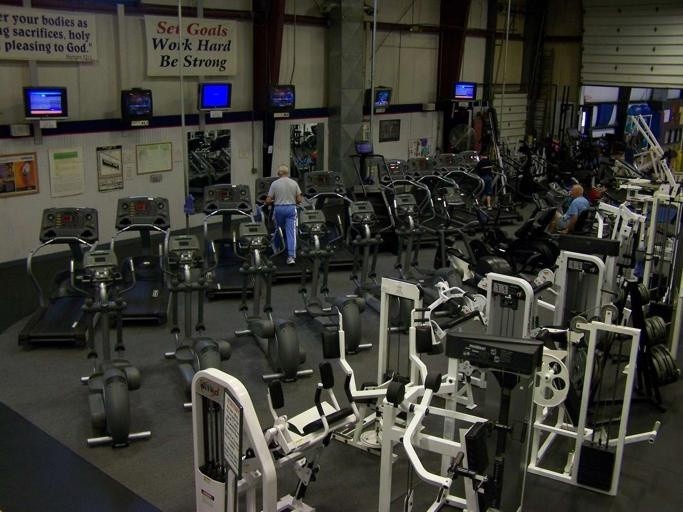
[197,82,232,113]
[270,85,295,110]
[454,82,477,100]
[22,86,69,120]
[365,85,392,109]
[121,87,153,120]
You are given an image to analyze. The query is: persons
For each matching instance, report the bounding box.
[595,177,623,235]
[547,184,590,235]
[477,139,493,212]
[265,166,302,267]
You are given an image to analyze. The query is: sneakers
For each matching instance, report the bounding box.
[286,257,295,265]
[272,244,280,254]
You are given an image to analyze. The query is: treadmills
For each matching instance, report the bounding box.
[19,206,101,349]
[347,152,524,247]
[299,170,362,271]
[202,183,264,302]
[105,196,170,326]
[254,177,307,284]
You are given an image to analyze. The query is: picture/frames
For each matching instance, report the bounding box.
[0,151,40,198]
[135,142,173,175]
